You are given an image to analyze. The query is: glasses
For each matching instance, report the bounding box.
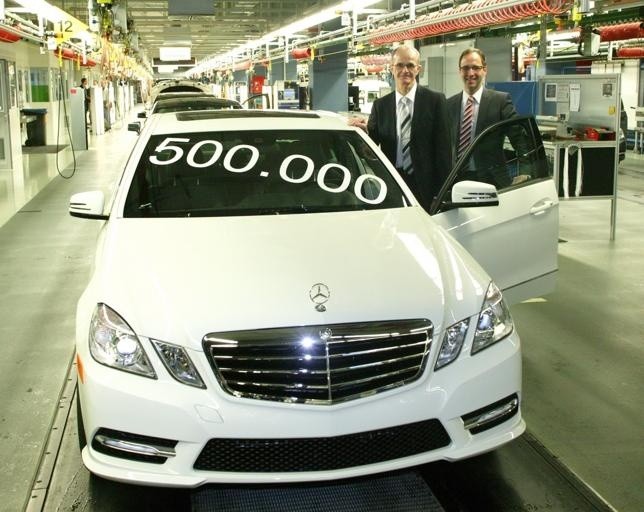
[461,66,484,71]
[392,64,419,69]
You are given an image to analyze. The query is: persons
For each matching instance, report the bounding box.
[348,45,450,213]
[447,48,533,191]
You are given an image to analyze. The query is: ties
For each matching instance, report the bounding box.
[400,97,415,175]
[458,95,473,166]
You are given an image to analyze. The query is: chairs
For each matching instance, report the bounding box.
[269,146,354,187]
[146,145,233,211]
